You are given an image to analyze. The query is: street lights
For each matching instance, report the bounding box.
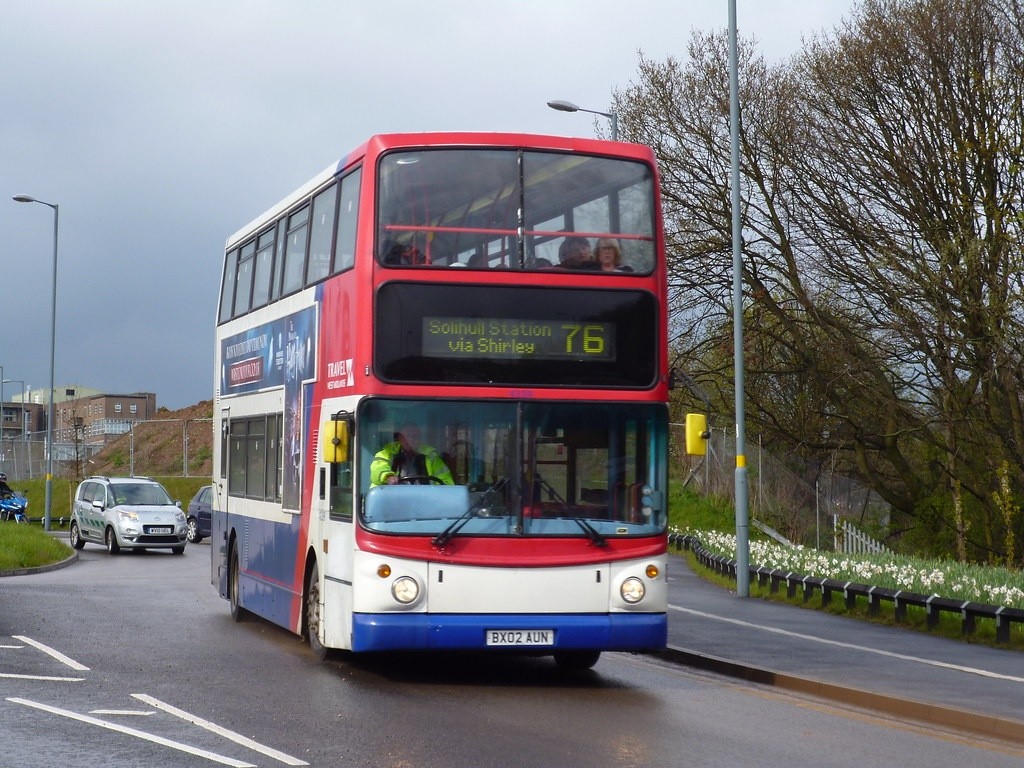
[545,96,620,517]
[2,379,26,478]
[9,192,61,532]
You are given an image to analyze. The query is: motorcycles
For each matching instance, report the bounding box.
[0,488,30,526]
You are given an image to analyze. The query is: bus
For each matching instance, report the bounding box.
[207,129,714,679]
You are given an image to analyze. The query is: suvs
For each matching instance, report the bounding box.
[68,474,189,555]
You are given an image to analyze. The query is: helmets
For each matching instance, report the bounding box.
[0,472,7,482]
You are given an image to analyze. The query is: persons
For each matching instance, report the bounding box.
[581,238,634,272]
[369,421,455,490]
[467,253,552,267]
[0,471,14,500]
[553,237,593,267]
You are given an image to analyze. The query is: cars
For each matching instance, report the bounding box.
[185,485,213,543]
